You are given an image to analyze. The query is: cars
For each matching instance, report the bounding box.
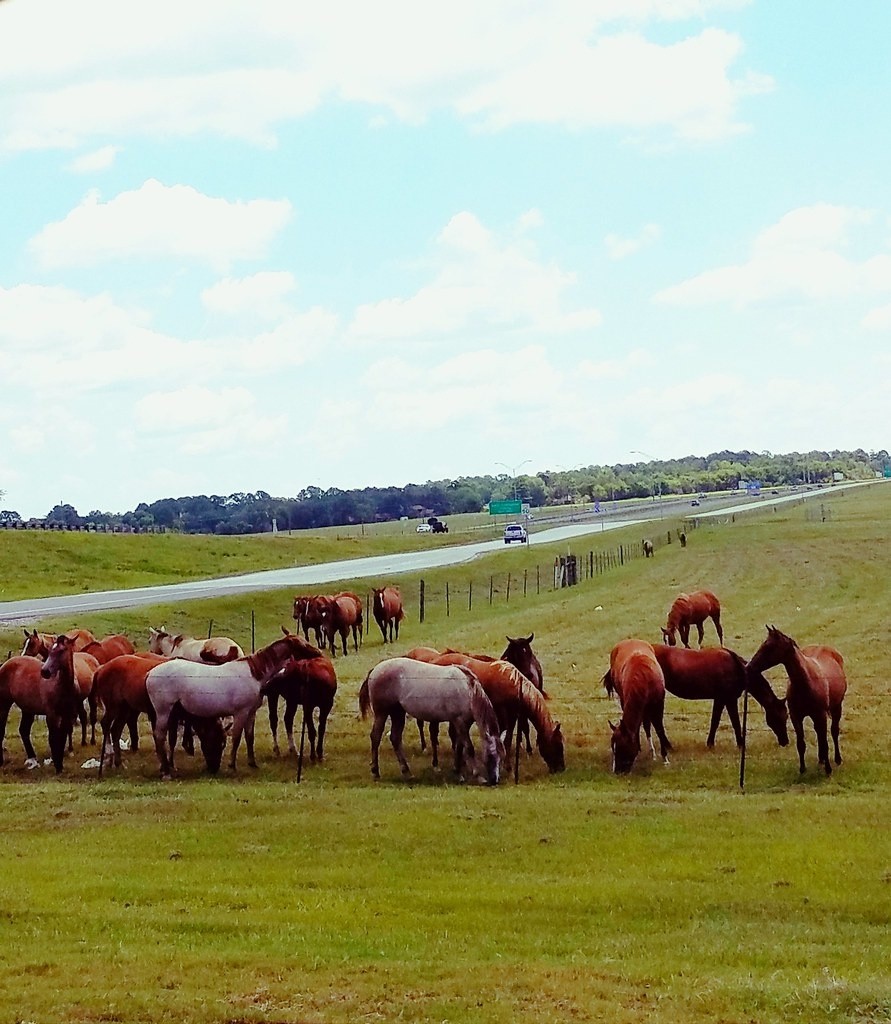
[416,524,431,532]
[0,510,21,527]
[699,494,707,499]
[432,521,448,533]
[691,500,700,506]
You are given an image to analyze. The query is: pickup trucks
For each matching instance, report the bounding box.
[503,525,527,544]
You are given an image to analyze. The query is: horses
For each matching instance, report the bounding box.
[0,585,851,789]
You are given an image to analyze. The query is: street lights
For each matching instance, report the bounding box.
[495,460,532,500]
[630,451,654,501]
